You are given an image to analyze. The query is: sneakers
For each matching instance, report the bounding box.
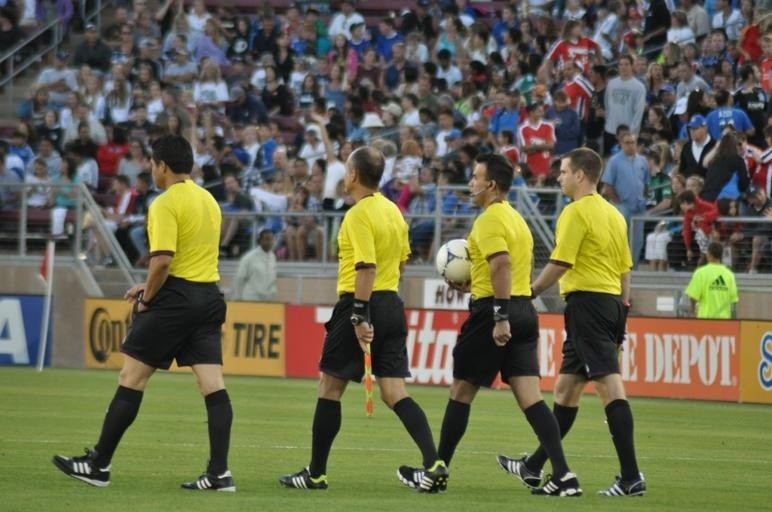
[493,450,544,490]
[176,466,238,494]
[414,457,451,495]
[277,464,331,490]
[593,467,648,498]
[49,445,113,487]
[528,469,586,499]
[396,461,451,491]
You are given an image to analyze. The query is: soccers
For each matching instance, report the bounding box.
[437,239,471,282]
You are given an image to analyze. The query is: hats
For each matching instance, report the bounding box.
[687,114,707,129]
[659,86,673,95]
[380,102,403,116]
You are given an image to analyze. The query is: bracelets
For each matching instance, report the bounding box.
[350,296,371,323]
[492,296,511,317]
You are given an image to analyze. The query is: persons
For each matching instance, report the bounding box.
[683,240,740,318]
[494,147,649,498]
[50,134,239,493]
[276,146,448,494]
[393,152,583,497]
[227,225,280,301]
[0,0,771,276]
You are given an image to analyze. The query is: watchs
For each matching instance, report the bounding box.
[491,311,510,322]
[349,314,366,327]
[137,292,151,307]
